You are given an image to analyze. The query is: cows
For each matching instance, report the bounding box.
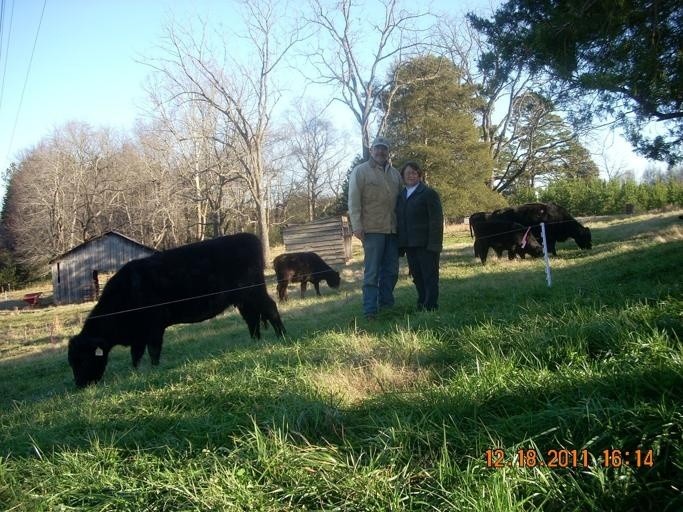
[469,202,592,267]
[67,232,289,390]
[273,251,341,302]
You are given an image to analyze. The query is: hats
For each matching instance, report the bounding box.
[372,137,390,150]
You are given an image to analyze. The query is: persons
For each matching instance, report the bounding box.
[392,160,445,314]
[348,136,403,323]
